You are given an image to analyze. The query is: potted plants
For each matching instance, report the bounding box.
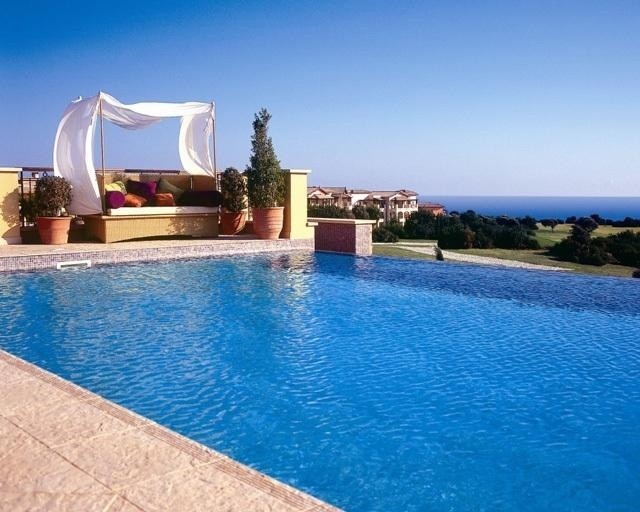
[220,167,248,234]
[34,176,72,244]
[19,198,41,244]
[242,108,287,240]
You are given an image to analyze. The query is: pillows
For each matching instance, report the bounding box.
[157,178,184,200]
[127,180,156,199]
[104,180,128,196]
[124,193,147,207]
[155,193,176,206]
[178,190,217,205]
[105,191,125,209]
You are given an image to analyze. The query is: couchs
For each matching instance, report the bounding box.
[78,173,219,244]
